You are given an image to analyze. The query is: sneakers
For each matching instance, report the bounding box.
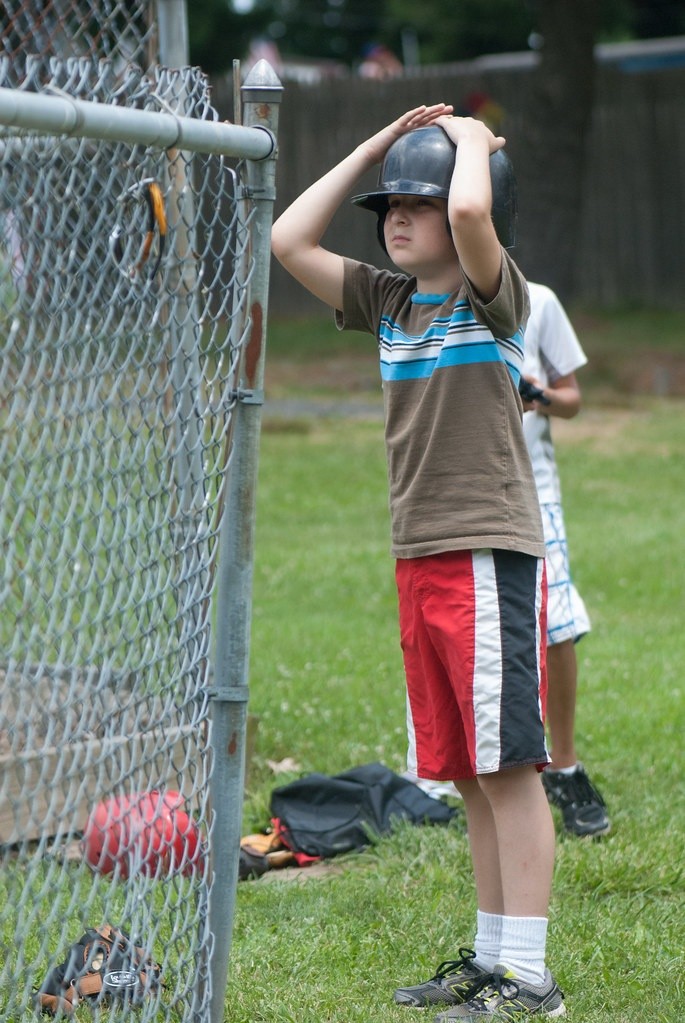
[542,761,610,839]
[394,948,490,1010]
[434,963,566,1023]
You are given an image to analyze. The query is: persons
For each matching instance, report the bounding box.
[271,101,569,1023]
[519,278,612,838]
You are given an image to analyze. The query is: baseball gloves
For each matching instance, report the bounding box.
[30,923,165,1021]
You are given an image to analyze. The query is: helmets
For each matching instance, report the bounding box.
[349,125,518,251]
[84,787,202,877]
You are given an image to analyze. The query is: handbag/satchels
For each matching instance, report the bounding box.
[271,762,454,861]
